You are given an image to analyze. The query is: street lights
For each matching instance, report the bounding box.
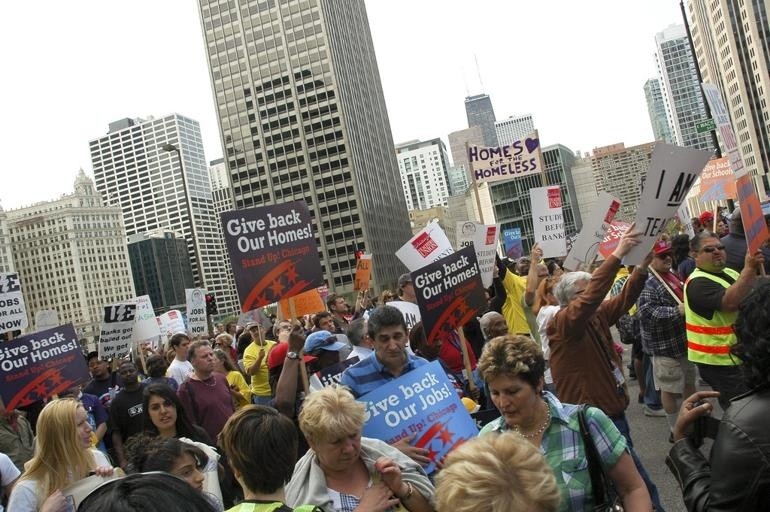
[161,143,215,341]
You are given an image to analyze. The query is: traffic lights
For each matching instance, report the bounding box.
[205,293,215,316]
[211,292,218,316]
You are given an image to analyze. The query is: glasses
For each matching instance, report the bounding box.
[311,335,338,353]
[701,246,726,252]
[369,333,408,343]
[707,218,713,223]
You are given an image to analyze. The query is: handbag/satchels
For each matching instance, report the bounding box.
[578,403,660,512]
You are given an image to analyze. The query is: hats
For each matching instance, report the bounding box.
[303,330,347,354]
[699,211,713,225]
[655,240,674,256]
[246,320,263,331]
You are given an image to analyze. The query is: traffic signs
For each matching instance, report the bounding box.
[693,118,719,133]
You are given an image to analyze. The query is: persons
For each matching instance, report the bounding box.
[1,205,770,512]
